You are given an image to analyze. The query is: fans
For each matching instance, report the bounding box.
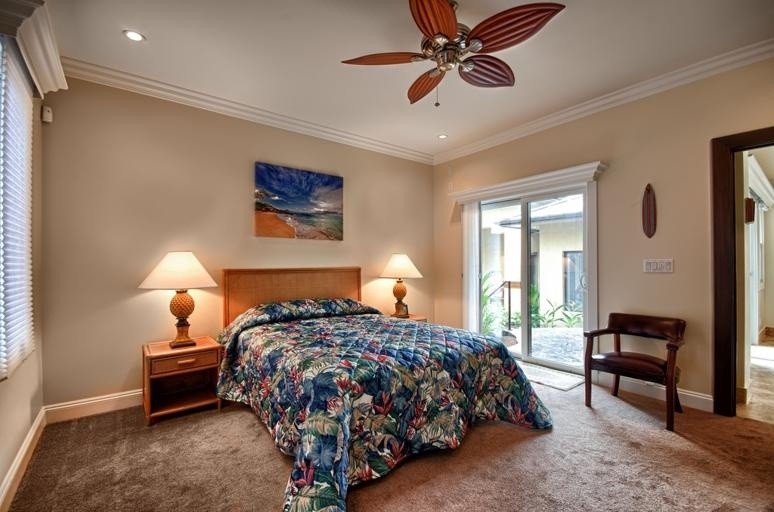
[340,0,566,106]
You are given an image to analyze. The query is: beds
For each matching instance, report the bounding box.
[221,266,504,486]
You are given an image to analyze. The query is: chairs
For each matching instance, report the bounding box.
[583,312,687,431]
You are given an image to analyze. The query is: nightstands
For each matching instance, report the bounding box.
[141,335,222,426]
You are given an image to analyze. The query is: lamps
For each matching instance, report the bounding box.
[137,251,218,349]
[378,253,424,318]
